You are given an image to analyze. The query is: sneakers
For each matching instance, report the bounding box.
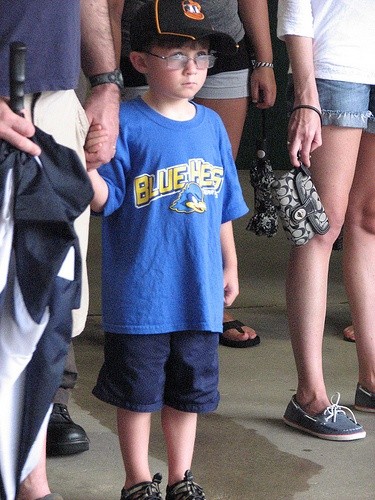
[47,404,88,456]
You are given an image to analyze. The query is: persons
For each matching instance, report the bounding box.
[108,0,278,349]
[87,1,242,500]
[0,0,122,457]
[274,0,375,441]
[0,95,61,500]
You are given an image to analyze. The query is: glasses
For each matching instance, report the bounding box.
[138,50,217,70]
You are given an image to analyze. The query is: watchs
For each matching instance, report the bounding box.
[90,68,124,88]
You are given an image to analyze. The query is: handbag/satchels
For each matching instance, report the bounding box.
[269,105,330,247]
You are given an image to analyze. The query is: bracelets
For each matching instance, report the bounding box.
[251,60,274,69]
[289,103,323,126]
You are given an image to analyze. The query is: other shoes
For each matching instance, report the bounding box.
[283,393,366,441]
[355,383,375,412]
[164,469,206,500]
[120,473,166,500]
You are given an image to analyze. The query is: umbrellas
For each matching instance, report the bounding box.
[246,98,278,238]
[0,42,94,500]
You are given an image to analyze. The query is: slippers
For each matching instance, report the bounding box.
[219,320,261,348]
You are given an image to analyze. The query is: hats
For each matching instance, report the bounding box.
[130,0,239,57]
